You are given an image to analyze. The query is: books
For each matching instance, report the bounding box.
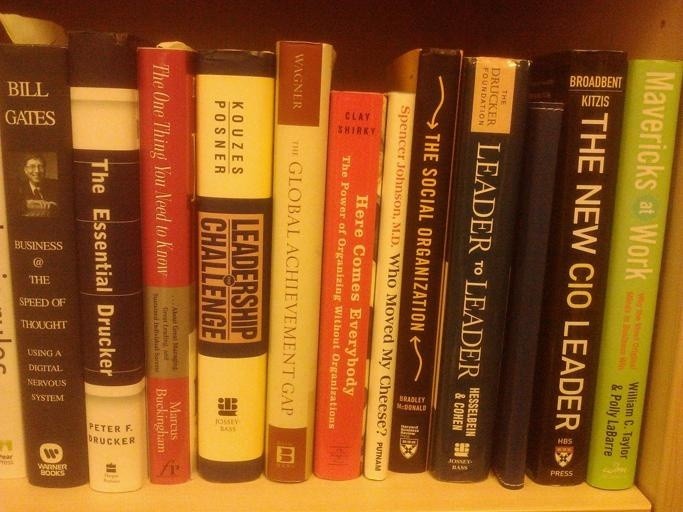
[2,12,682,490]
